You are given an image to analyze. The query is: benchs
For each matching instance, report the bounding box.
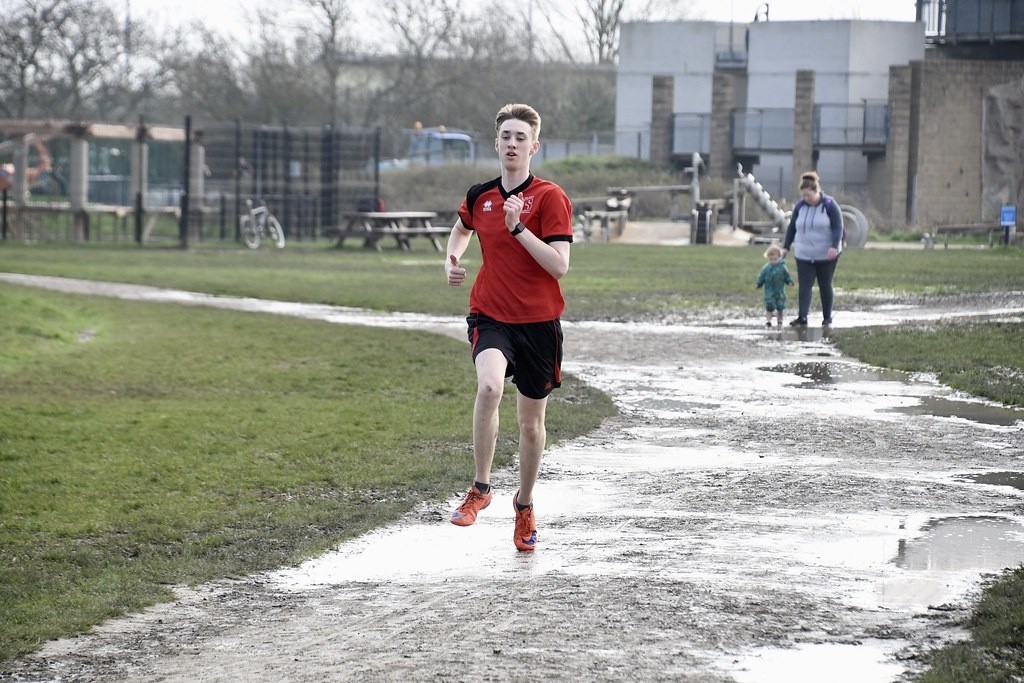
[934,222,1008,248]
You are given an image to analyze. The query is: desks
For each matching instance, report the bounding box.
[335,210,445,254]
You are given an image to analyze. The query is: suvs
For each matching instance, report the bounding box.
[366,128,474,175]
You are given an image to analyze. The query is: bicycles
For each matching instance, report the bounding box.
[238,193,286,250]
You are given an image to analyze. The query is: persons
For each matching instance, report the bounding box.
[779,172,846,326]
[755,243,795,329]
[443,103,573,550]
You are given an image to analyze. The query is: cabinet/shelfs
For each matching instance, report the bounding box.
[330,225,454,244]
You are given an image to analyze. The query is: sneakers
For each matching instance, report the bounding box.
[450,484,492,527]
[513,489,538,550]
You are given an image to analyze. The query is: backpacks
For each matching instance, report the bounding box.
[797,197,847,242]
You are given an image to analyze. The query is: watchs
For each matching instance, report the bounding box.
[510,222,525,236]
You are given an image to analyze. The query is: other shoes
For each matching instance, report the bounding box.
[822,318,832,325]
[790,318,807,326]
[777,323,782,327]
[766,322,771,327]
